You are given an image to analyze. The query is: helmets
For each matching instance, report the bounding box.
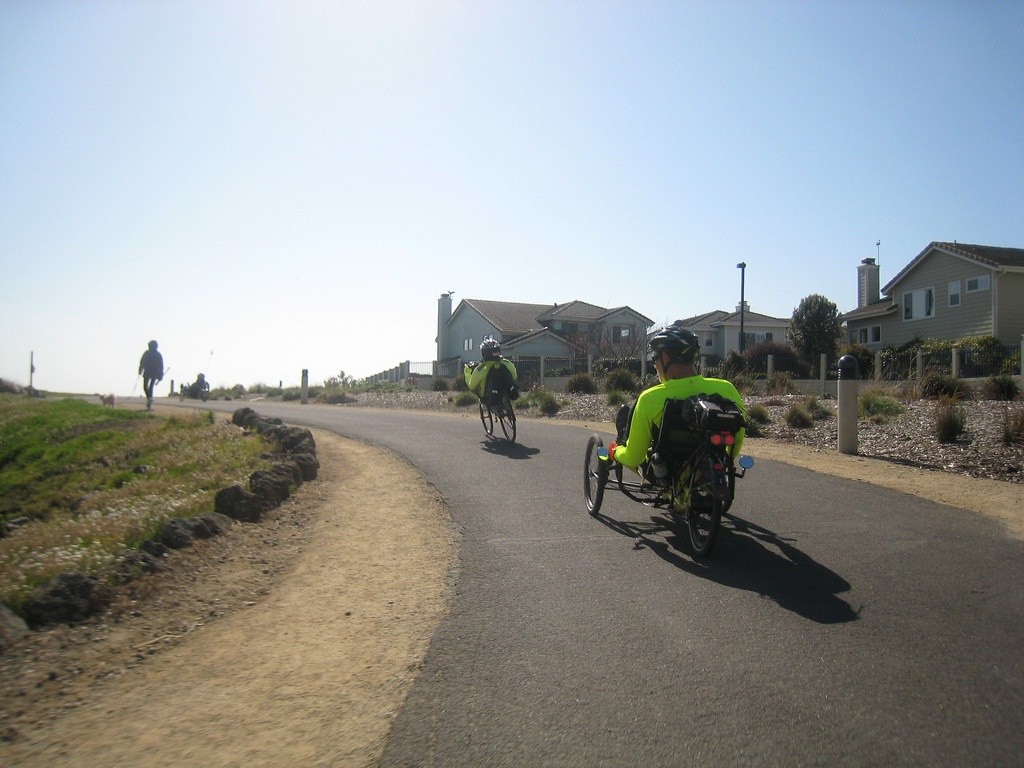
[479,338,500,351]
[649,326,700,357]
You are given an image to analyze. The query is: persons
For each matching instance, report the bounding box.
[607,324,746,488]
[464,338,517,411]
[138,340,163,407]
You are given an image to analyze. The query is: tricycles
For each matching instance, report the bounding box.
[179,384,211,402]
[583,392,755,558]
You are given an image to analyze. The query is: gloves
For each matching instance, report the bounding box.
[608,440,627,460]
[464,364,472,374]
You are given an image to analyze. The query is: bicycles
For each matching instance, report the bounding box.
[479,389,520,442]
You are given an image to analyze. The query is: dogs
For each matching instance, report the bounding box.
[100,393,116,408]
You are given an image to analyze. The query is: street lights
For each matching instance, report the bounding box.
[736,262,747,355]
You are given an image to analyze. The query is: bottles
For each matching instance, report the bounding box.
[651,453,667,479]
